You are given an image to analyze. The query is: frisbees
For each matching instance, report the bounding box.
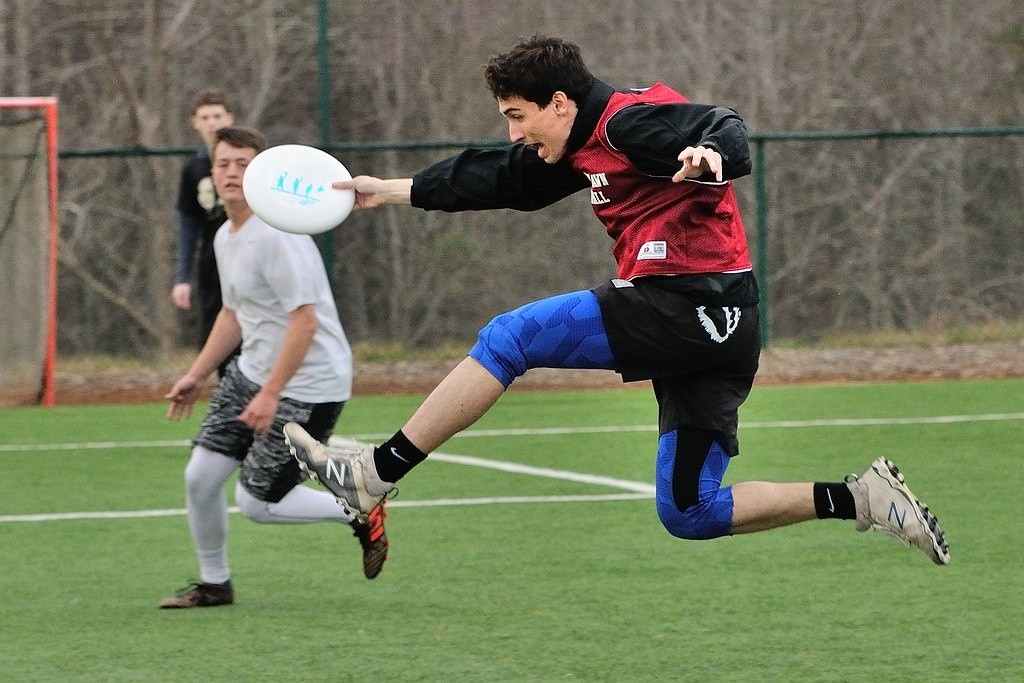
[242,142,357,236]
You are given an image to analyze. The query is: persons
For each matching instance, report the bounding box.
[168,89,242,389]
[281,32,952,568]
[163,124,392,611]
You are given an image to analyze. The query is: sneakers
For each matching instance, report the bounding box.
[282,421,398,525]
[158,579,235,608]
[844,456,950,566]
[349,499,389,580]
